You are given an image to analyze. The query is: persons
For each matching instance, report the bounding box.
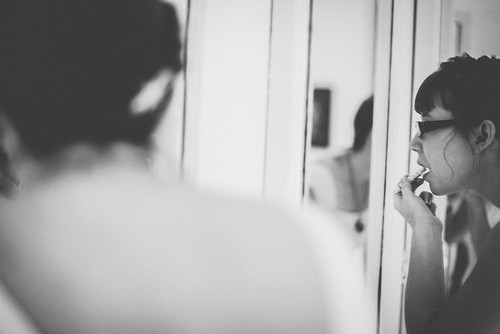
[391,53,499,334]
[0,0,330,333]
[305,93,375,211]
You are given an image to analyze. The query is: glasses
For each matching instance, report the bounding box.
[415,119,460,138]
